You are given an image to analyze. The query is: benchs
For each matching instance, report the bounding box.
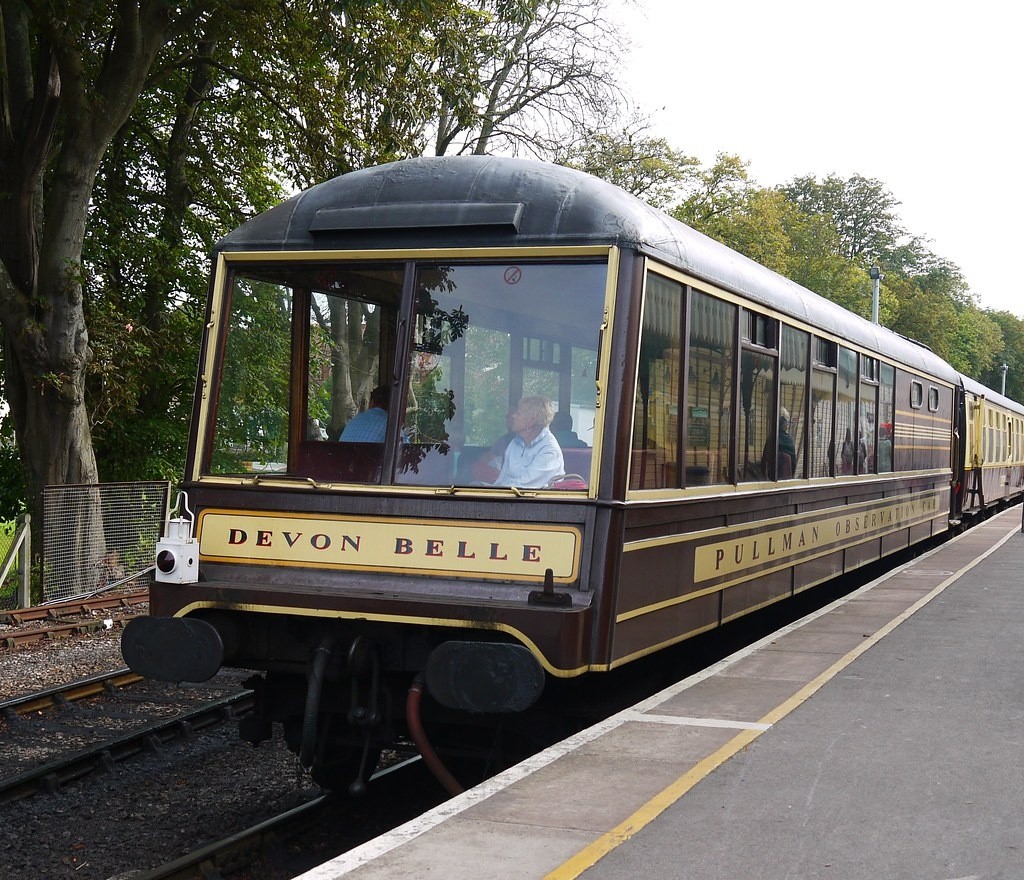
[296,439,716,494]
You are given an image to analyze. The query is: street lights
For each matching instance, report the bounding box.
[999,364,1009,396]
[867,267,886,323]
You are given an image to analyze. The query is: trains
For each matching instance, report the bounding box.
[118,152,1024,802]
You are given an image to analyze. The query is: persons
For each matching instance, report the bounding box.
[468,394,588,489]
[840,427,893,475]
[762,416,798,479]
[340,384,408,444]
